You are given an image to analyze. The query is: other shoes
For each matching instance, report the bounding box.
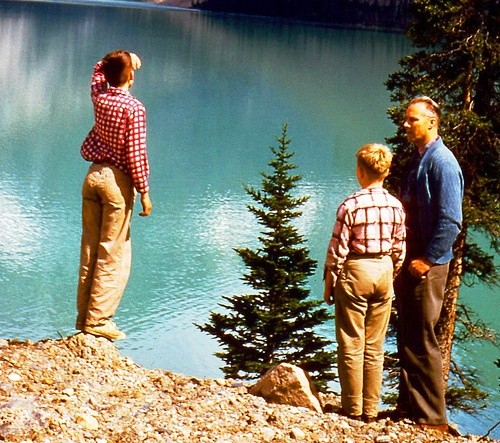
[364,416,376,426]
[325,404,361,421]
[83,324,127,341]
[415,423,448,432]
[396,406,410,420]
[75,321,117,331]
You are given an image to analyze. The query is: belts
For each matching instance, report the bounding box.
[346,253,382,260]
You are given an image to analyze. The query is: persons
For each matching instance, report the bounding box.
[321,144,409,426]
[72,50,153,344]
[386,95,466,430]
